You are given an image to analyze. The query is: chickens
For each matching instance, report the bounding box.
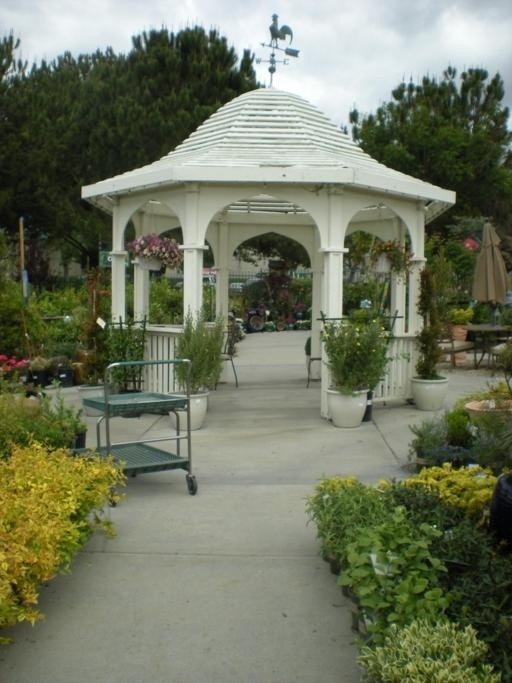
[268,13,293,48]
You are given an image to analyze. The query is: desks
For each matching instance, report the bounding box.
[462,324,512,370]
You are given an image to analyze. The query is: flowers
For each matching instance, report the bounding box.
[320,313,410,398]
[286,315,296,323]
[291,303,305,312]
[365,238,419,286]
[127,233,183,269]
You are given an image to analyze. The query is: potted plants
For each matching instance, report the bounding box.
[26,356,76,387]
[77,332,115,417]
[409,265,450,411]
[445,307,475,364]
[167,302,227,431]
[64,408,88,449]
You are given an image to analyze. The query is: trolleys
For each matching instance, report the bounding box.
[70,355,199,504]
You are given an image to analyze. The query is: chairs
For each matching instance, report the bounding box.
[437,338,456,368]
[207,313,238,390]
[487,327,512,377]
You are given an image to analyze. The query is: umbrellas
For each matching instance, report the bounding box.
[470,219,511,323]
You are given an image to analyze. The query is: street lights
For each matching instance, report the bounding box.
[206,268,217,323]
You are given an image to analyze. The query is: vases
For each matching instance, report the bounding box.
[136,254,163,272]
[324,388,369,428]
[296,311,304,320]
[464,399,512,429]
[368,249,392,274]
[288,323,295,329]
